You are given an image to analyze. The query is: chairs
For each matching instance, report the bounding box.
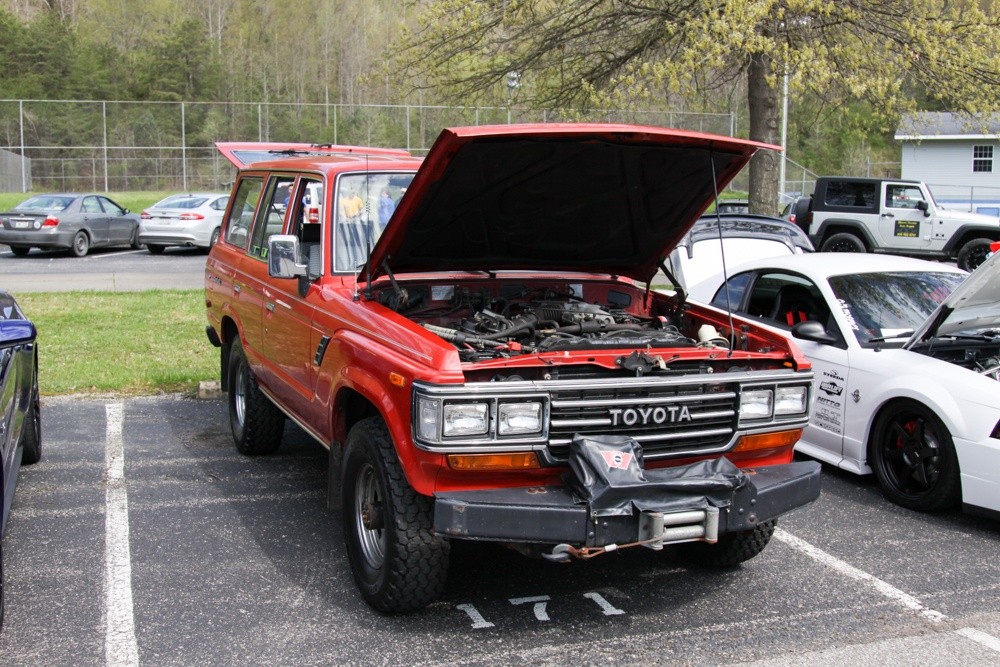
[771,284,826,327]
[303,223,368,271]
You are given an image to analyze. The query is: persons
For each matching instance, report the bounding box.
[283,185,405,268]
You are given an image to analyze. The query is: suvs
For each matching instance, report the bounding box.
[794,175,1000,276]
[204,120,822,617]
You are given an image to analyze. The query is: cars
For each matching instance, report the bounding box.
[655,213,1000,513]
[0,290,43,630]
[779,200,798,225]
[0,193,144,257]
[139,193,258,253]
[265,182,373,234]
[715,199,749,214]
